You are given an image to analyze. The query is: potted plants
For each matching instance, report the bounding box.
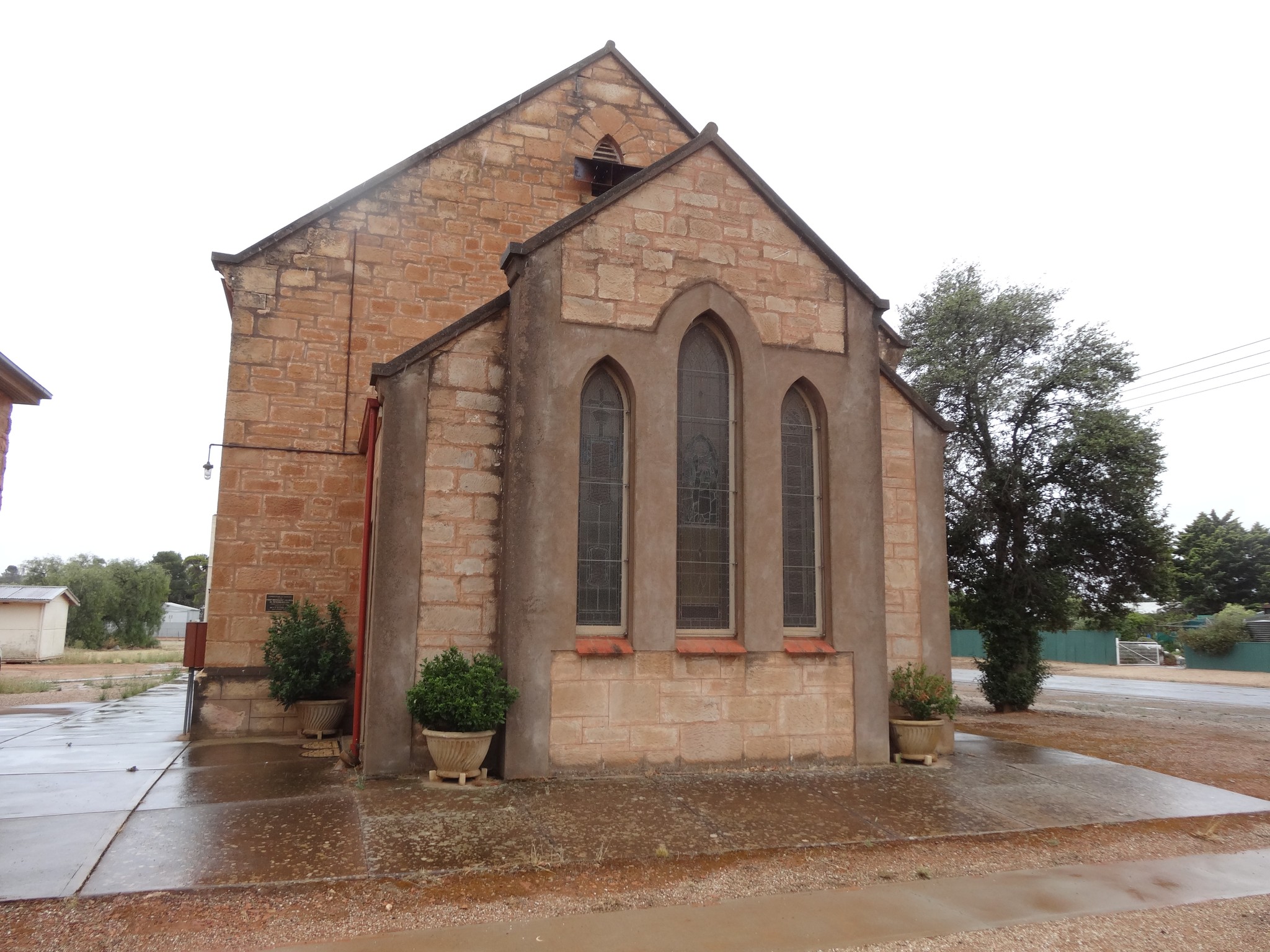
[885,658,965,762]
[402,643,522,778]
[260,596,356,742]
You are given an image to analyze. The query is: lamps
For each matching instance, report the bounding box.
[202,444,221,480]
